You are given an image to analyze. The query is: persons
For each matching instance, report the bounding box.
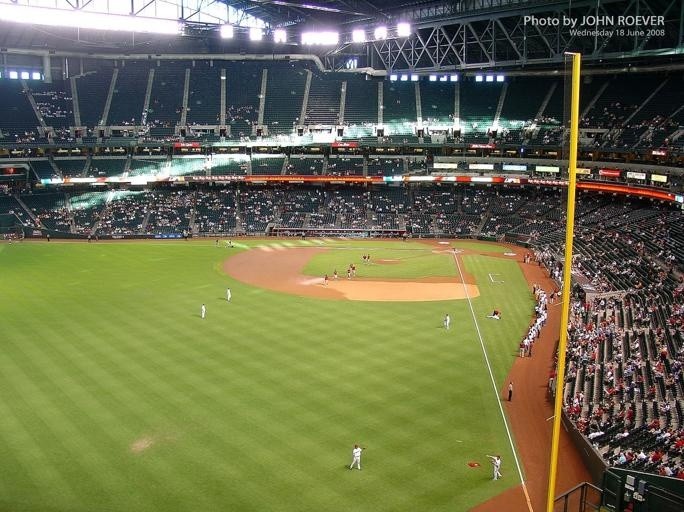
[1,90,684,512]
